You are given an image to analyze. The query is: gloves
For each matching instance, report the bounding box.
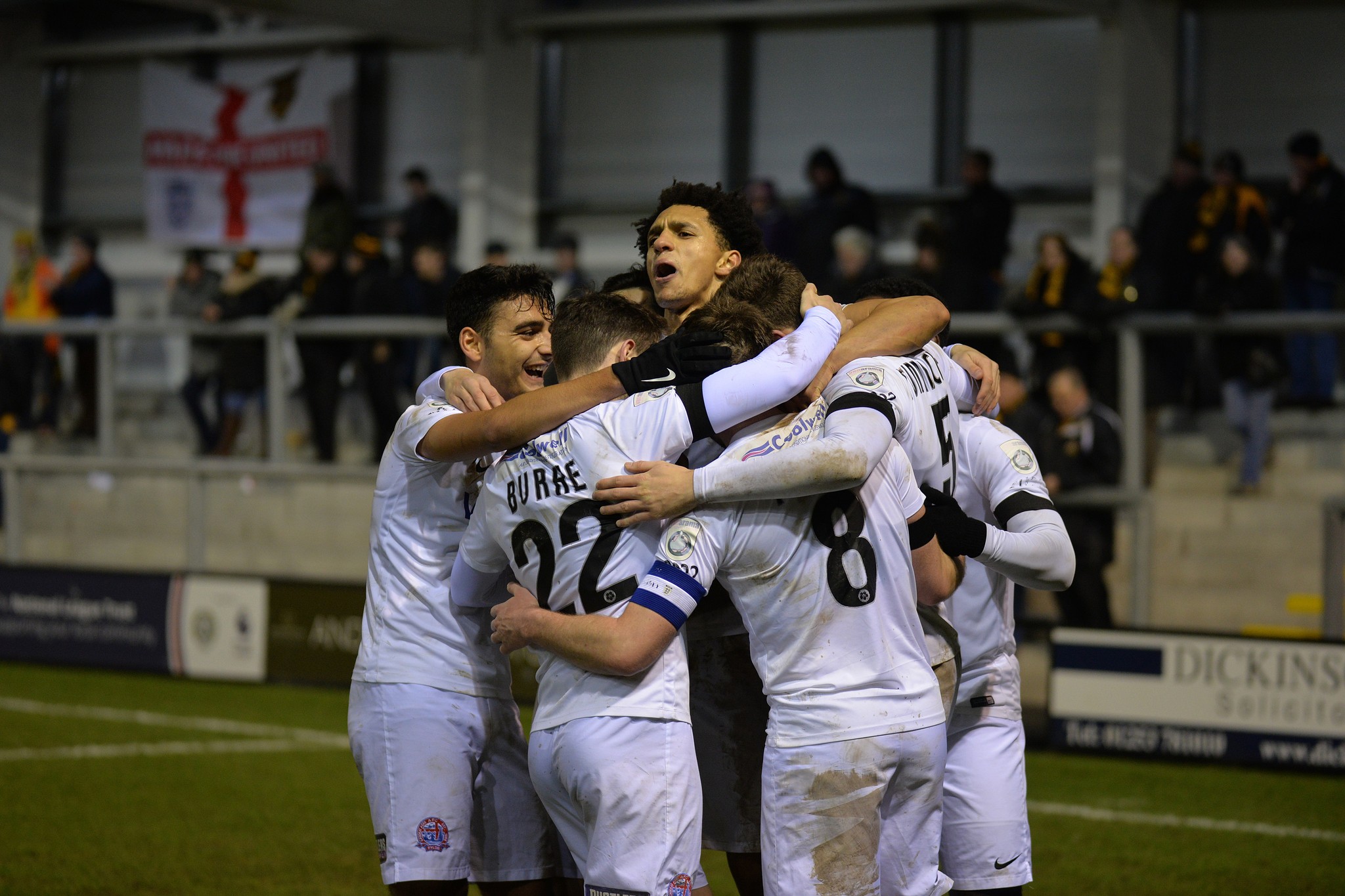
[611,321,734,399]
[920,481,986,558]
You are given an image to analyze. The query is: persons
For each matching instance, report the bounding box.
[51,221,121,443]
[344,259,709,896]
[597,263,664,316]
[166,105,1342,660]
[405,178,951,892]
[0,224,68,439]
[447,283,856,895]
[914,406,1080,891]
[489,296,952,896]
[588,252,1005,737]
